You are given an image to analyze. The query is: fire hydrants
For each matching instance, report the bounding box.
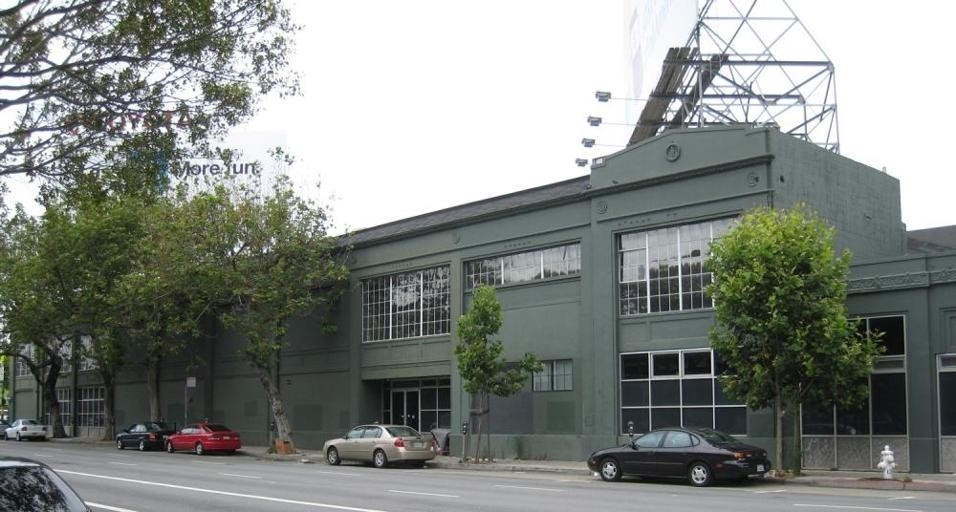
[876,444,897,480]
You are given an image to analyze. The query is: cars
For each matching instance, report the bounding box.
[164,423,243,455]
[0,456,92,512]
[321,422,437,467]
[588,425,773,486]
[0,419,10,437]
[4,419,48,442]
[111,420,175,453]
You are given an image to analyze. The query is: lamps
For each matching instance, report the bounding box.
[574,86,696,167]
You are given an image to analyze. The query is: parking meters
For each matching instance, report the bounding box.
[459,418,471,460]
[626,419,637,442]
